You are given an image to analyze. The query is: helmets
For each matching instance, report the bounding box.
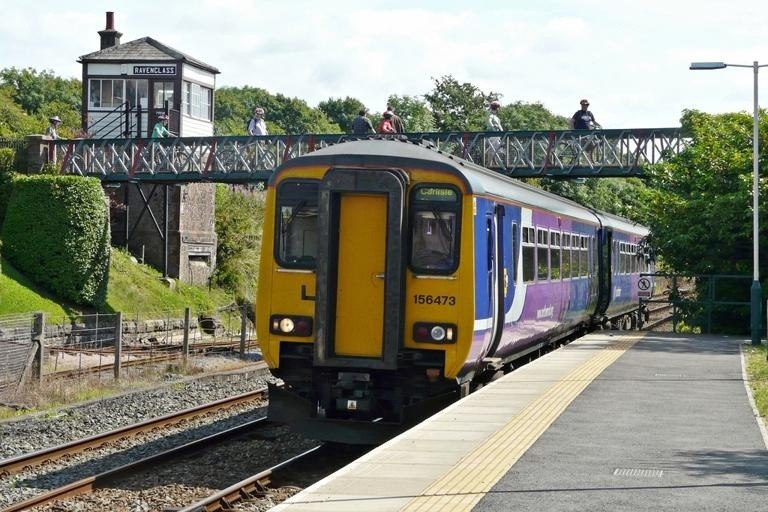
[157,115,170,120]
[383,111,394,117]
[255,108,264,115]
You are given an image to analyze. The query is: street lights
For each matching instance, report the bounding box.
[689,61,768,345]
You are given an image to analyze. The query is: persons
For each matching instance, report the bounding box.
[46,116,61,140]
[152,115,178,138]
[352,107,404,133]
[487,104,503,141]
[571,100,600,153]
[248,108,272,144]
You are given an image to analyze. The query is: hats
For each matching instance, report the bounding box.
[580,99,589,105]
[49,115,61,122]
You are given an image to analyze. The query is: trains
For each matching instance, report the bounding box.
[256,137,656,445]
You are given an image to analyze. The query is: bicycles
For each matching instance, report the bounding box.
[548,125,616,165]
[60,145,85,173]
[453,137,519,167]
[216,140,276,171]
[141,146,190,171]
